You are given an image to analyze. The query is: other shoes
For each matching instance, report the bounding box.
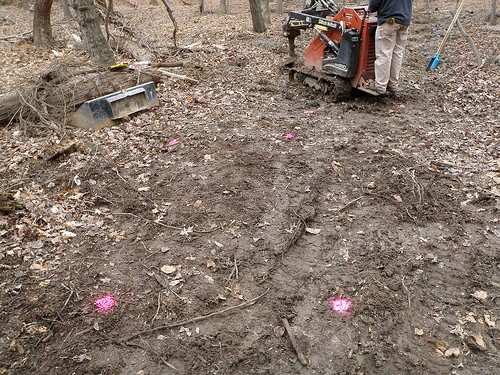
[368,79,385,94]
[387,85,397,92]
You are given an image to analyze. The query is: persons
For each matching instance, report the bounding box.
[366,0,413,94]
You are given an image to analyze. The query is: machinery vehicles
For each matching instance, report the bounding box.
[281,0,395,103]
